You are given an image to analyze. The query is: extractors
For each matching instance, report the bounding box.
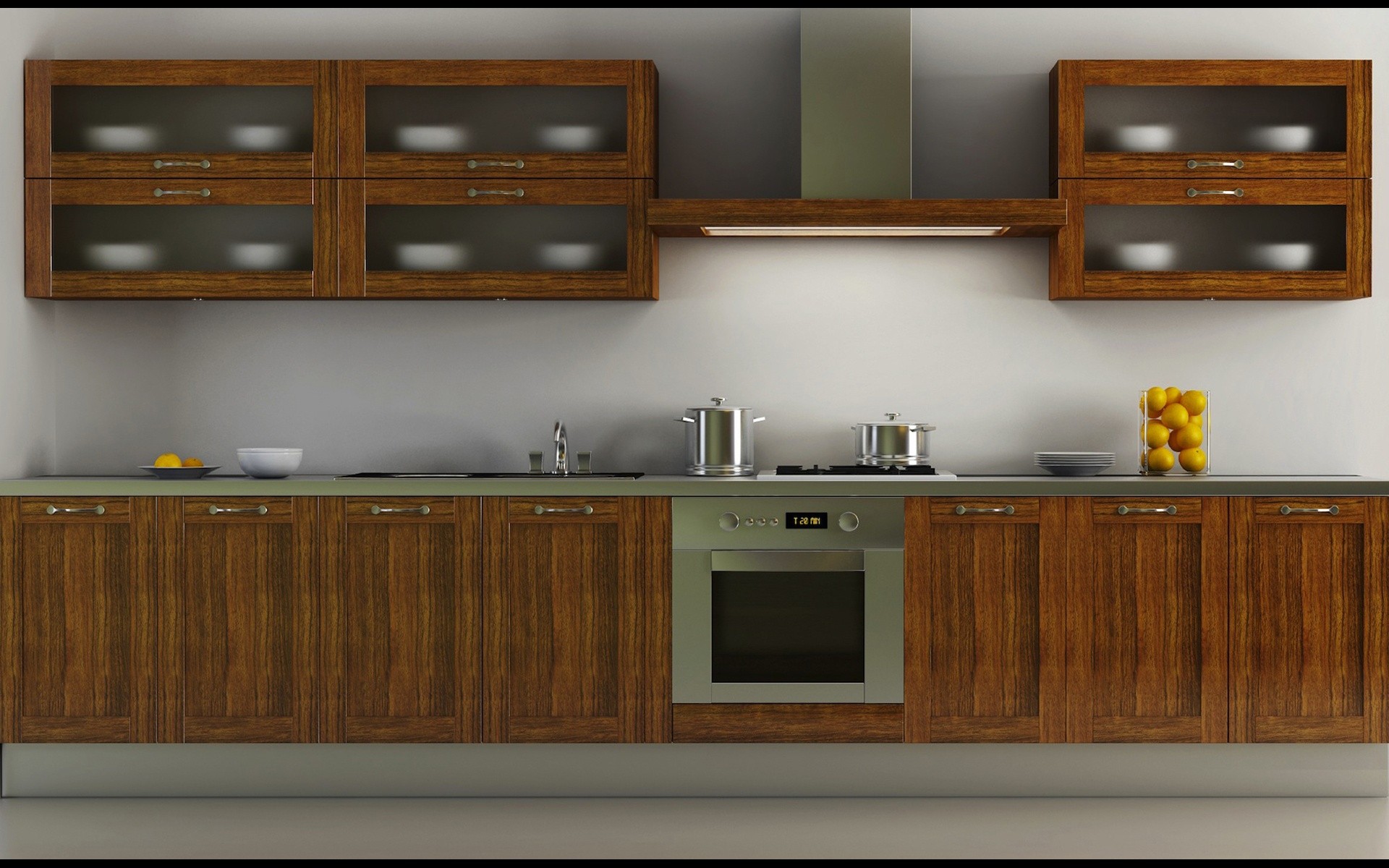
[699,8,1010,237]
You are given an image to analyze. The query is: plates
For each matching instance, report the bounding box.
[138,466,223,479]
[1033,451,1115,476]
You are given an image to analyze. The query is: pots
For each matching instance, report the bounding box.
[674,397,766,476]
[850,413,936,464]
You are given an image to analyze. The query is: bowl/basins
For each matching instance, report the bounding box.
[1246,126,1315,153]
[224,241,295,270]
[393,125,471,152]
[225,125,297,152]
[390,244,479,269]
[530,242,602,270]
[1106,123,1175,151]
[81,244,165,271]
[236,448,302,477]
[1243,244,1314,269]
[1113,243,1179,270]
[532,123,604,151]
[81,126,160,152]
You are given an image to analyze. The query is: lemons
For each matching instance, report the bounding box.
[182,457,203,466]
[1139,387,1206,473]
[154,453,181,467]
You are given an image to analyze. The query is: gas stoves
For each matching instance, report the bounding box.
[756,464,957,480]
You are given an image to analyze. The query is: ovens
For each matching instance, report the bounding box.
[671,495,905,705]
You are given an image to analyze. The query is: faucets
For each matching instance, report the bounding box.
[553,422,571,475]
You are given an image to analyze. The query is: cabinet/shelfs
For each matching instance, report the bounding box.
[1050,58,1373,302]
[0,494,672,743]
[23,56,661,301]
[903,495,1388,744]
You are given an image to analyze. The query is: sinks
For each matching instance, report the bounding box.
[332,471,478,478]
[466,471,646,481]
